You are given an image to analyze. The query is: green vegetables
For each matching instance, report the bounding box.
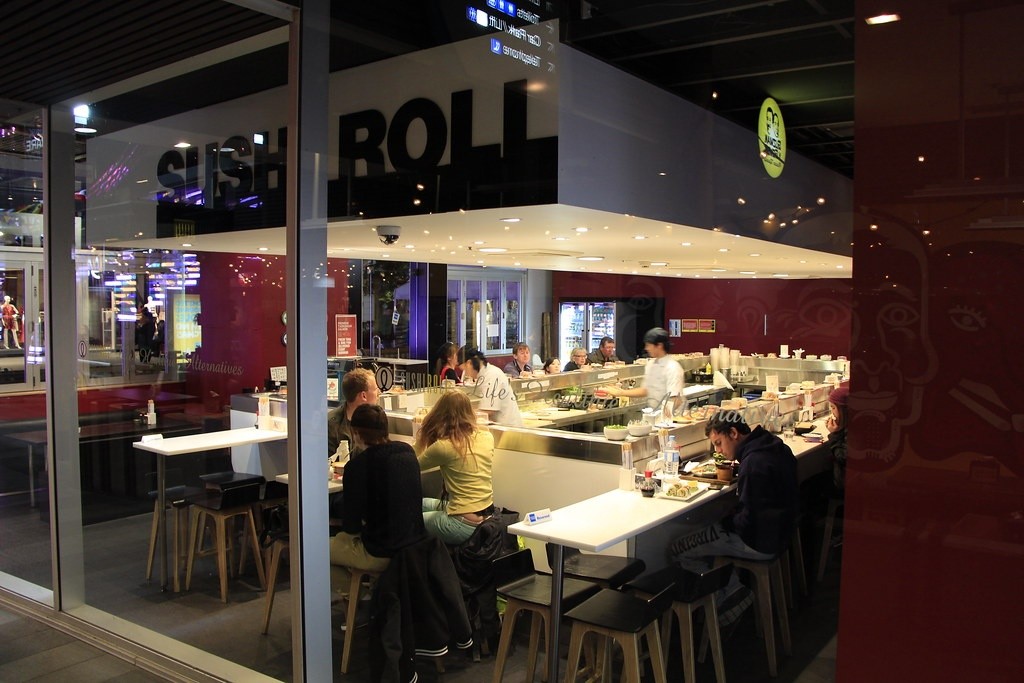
[565,386,581,394]
[606,424,626,429]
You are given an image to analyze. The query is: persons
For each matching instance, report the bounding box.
[668,411,801,641]
[412,391,497,622]
[457,342,523,428]
[812,387,848,548]
[329,404,427,632]
[135,306,165,364]
[440,342,465,384]
[540,356,561,374]
[565,336,620,372]
[0,295,23,351]
[605,327,689,419]
[503,342,533,378]
[328,367,382,458]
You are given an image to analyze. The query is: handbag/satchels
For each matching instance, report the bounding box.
[268,504,288,539]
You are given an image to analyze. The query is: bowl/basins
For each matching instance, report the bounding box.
[627,423,652,436]
[785,354,844,392]
[332,462,346,477]
[794,425,817,436]
[604,426,629,440]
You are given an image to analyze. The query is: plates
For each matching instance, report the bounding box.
[657,480,711,500]
[655,405,747,429]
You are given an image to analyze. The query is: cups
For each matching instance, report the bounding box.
[643,414,655,427]
[710,347,740,384]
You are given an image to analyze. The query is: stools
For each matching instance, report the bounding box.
[144,454,844,683]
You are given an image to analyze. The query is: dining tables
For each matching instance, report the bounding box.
[131,422,439,683]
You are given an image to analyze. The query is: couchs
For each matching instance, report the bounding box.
[0,407,161,508]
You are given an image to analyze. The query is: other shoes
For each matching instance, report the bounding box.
[716,588,755,627]
[340,600,372,630]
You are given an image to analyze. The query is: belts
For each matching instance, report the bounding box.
[452,515,479,527]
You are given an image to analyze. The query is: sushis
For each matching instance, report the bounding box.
[665,483,691,497]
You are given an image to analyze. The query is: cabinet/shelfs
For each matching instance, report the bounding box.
[558,301,637,372]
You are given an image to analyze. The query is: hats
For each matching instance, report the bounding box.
[827,386,848,407]
[456,345,476,366]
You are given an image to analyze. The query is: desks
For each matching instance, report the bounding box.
[4,416,202,526]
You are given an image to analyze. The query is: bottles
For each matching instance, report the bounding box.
[783,428,794,443]
[339,440,350,462]
[664,435,679,479]
[640,471,654,497]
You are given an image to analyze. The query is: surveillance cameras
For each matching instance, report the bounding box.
[375,226,401,245]
